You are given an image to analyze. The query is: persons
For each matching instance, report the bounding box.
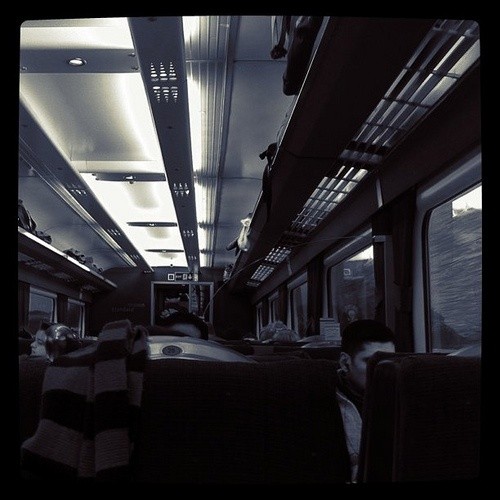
[334,319,399,487]
[159,312,209,343]
[29,319,79,362]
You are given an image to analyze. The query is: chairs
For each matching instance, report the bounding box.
[14,326,482,488]
